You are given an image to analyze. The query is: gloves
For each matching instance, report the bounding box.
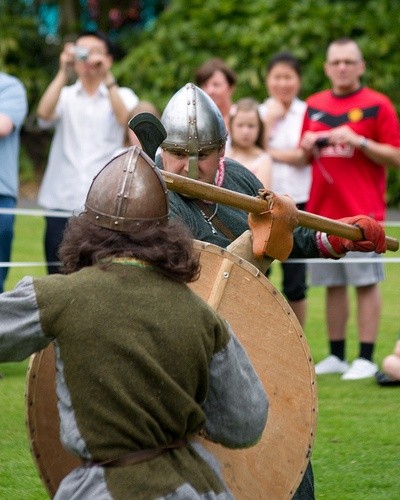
[247,190,299,263]
[316,214,387,260]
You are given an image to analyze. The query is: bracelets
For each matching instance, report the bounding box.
[359,136,367,150]
[106,81,118,92]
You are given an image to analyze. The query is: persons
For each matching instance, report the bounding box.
[255,53,314,338]
[0,148,272,499]
[0,71,30,299]
[223,99,272,194]
[35,37,143,281]
[195,62,238,155]
[146,84,318,499]
[299,38,400,383]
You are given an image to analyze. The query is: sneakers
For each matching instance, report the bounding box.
[314,354,347,375]
[342,357,379,380]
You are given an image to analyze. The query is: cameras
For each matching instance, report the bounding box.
[314,131,337,148]
[68,45,90,62]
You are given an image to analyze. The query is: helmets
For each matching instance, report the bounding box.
[82,144,170,234]
[157,81,229,154]
